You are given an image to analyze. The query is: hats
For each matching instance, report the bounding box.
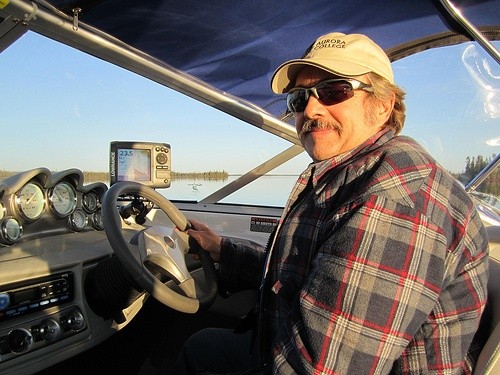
[271,32,394,95]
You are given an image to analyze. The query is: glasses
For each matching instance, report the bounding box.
[286,78,374,112]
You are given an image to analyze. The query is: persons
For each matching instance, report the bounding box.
[169,32,489,374]
